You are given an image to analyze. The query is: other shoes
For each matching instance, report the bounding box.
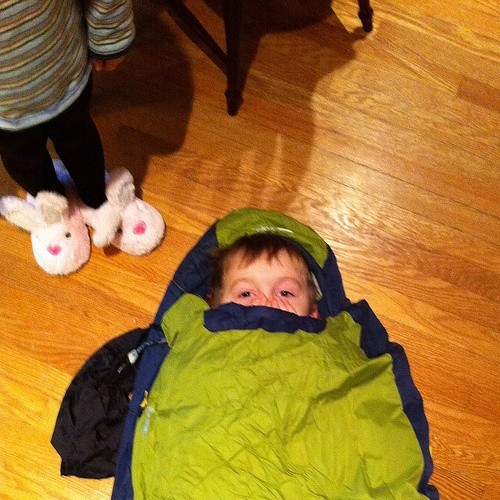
[76,166,165,255]
[1,160,89,276]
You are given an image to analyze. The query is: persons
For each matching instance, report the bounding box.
[109,207,440,500]
[0,0,169,279]
